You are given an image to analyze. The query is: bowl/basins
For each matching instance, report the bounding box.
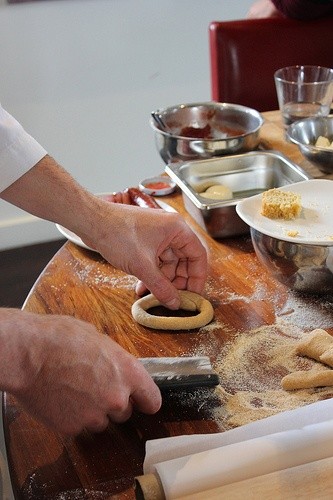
[285,114,333,174]
[248,221,333,295]
[163,146,315,238]
[150,101,263,159]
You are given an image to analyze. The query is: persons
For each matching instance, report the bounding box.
[0,108,208,434]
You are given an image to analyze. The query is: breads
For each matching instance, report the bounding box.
[262,189,302,220]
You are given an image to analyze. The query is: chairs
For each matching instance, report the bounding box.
[210,16,333,113]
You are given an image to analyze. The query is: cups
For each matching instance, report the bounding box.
[275,65,333,143]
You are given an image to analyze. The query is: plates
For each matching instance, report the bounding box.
[234,178,332,246]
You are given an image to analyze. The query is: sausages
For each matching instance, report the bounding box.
[128,187,162,210]
